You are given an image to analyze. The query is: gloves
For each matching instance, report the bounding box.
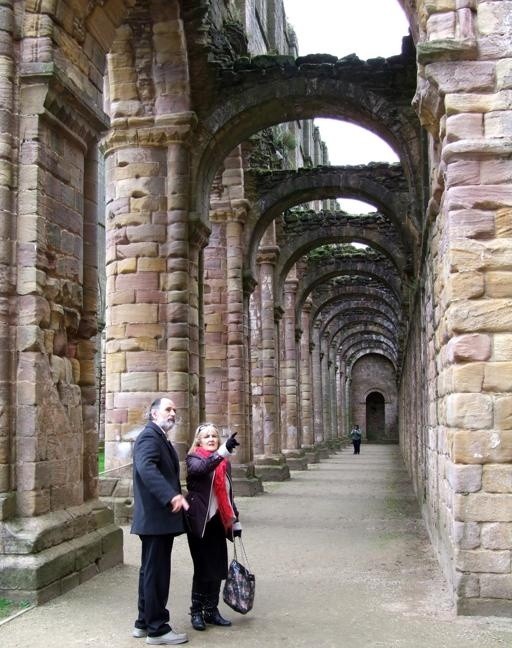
[233,530,242,539]
[226,432,239,454]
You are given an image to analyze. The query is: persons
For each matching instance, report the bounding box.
[349,424,362,455]
[182,421,245,632]
[128,396,192,647]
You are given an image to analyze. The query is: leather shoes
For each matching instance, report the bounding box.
[193,611,206,631]
[204,608,232,626]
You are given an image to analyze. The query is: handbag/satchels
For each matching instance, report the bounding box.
[223,560,254,614]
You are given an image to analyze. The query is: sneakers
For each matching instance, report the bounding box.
[133,628,188,645]
